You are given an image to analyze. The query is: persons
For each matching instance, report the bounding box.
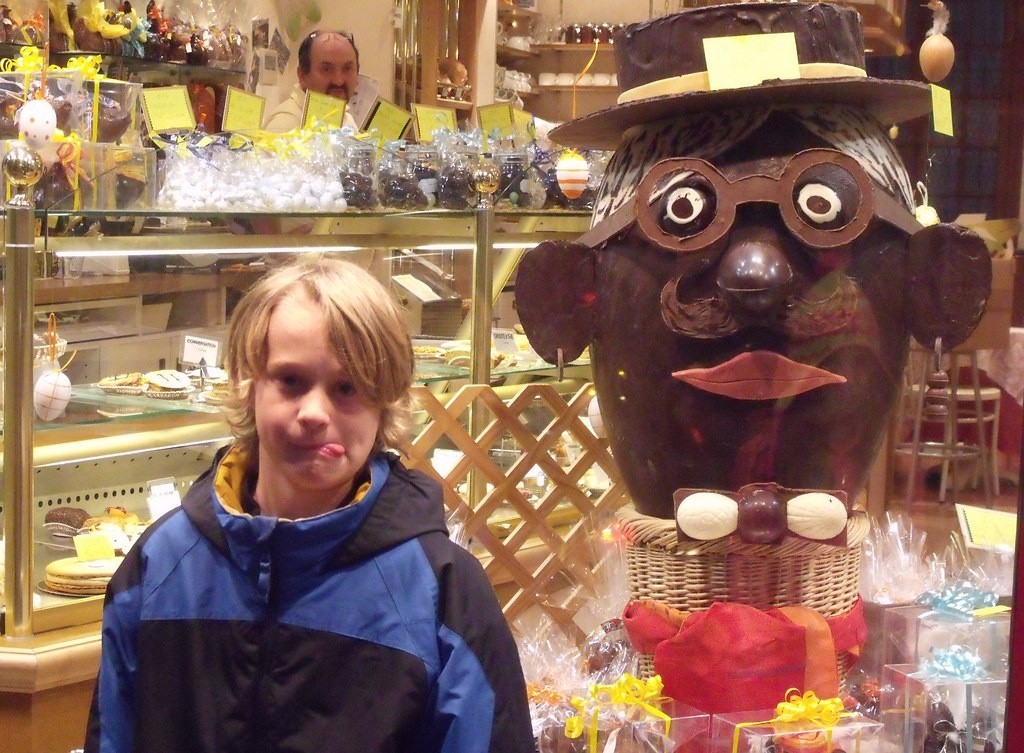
[265,28,359,134]
[512,5,994,551]
[81,252,537,753]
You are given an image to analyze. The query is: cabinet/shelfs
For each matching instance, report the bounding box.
[495,2,541,97]
[0,206,593,753]
[530,43,618,92]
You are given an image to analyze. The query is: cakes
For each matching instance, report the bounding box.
[43,555,125,595]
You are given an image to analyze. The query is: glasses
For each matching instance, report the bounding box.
[304,29,355,46]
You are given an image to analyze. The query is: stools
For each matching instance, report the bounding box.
[906,383,1002,505]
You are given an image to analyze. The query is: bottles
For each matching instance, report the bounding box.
[335,145,602,210]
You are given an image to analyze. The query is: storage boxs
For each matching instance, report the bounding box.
[711,688,885,753]
[0,46,156,210]
[879,645,1008,753]
[524,683,618,753]
[562,673,709,753]
[883,578,1010,663]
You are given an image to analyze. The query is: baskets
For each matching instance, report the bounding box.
[615,501,871,688]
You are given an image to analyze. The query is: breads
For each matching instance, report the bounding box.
[98,337,517,402]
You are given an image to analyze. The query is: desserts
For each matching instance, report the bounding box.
[44,507,153,557]
[11,99,598,208]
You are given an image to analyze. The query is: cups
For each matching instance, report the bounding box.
[535,19,627,45]
[538,72,619,86]
[497,21,534,110]
